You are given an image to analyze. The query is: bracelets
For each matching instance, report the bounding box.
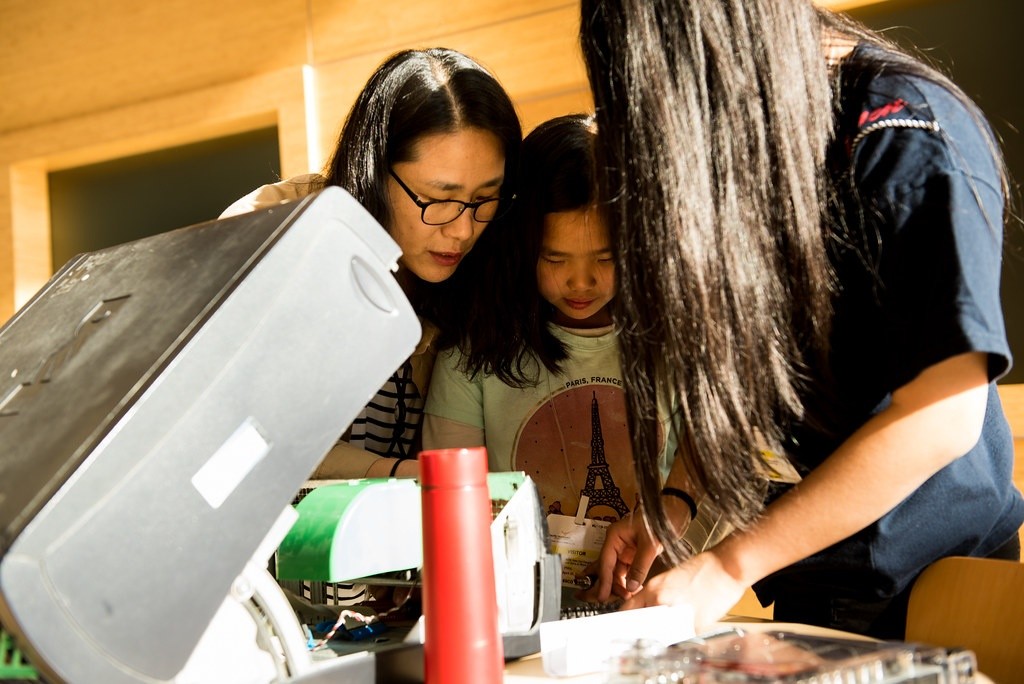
[389,459,406,477]
[661,488,698,521]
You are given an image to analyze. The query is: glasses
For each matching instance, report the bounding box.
[387,165,517,226]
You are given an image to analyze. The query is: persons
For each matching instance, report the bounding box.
[218,48,523,480]
[422,113,682,607]
[579,0,1023,644]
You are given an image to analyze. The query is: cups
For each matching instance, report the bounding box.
[418,445,504,684]
